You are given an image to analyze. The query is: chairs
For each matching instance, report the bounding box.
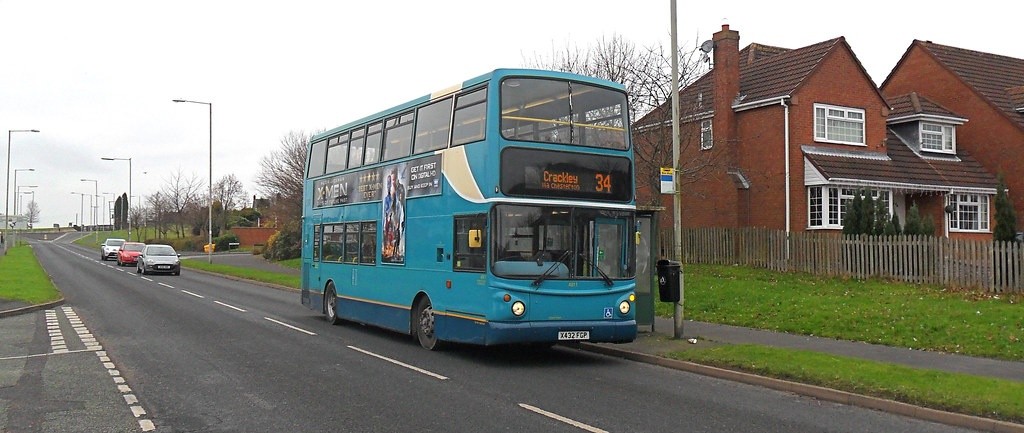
[324,254,359,264]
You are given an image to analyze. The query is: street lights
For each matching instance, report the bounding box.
[172,100,212,265]
[71,158,148,246]
[4,130,40,256]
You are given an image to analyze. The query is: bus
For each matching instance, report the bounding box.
[300,69,637,351]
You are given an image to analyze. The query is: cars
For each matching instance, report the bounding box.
[116,242,146,266]
[101,239,128,261]
[136,245,180,276]
